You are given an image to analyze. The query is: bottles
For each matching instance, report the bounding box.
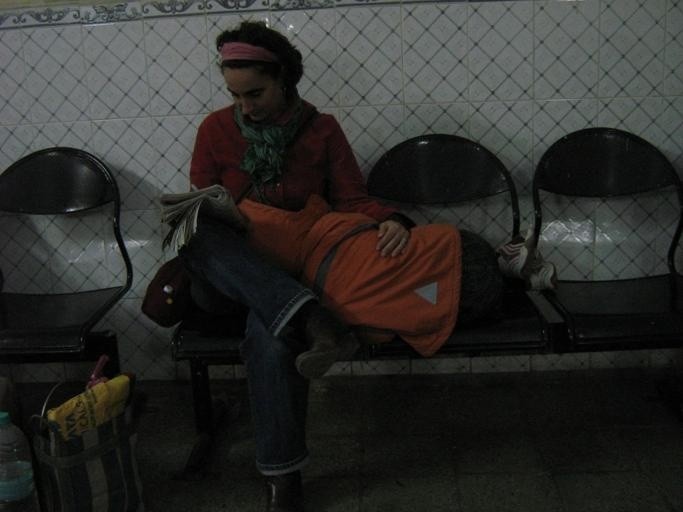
[0,410,42,512]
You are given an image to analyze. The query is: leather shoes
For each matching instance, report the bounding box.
[295,314,359,381]
[264,468,304,511]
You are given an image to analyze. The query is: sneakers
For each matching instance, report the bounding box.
[495,227,536,281]
[530,252,560,293]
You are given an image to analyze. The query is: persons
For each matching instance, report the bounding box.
[236,194,557,332]
[184,18,417,510]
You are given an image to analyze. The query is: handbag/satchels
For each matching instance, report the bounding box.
[141,254,189,328]
[28,380,145,511]
